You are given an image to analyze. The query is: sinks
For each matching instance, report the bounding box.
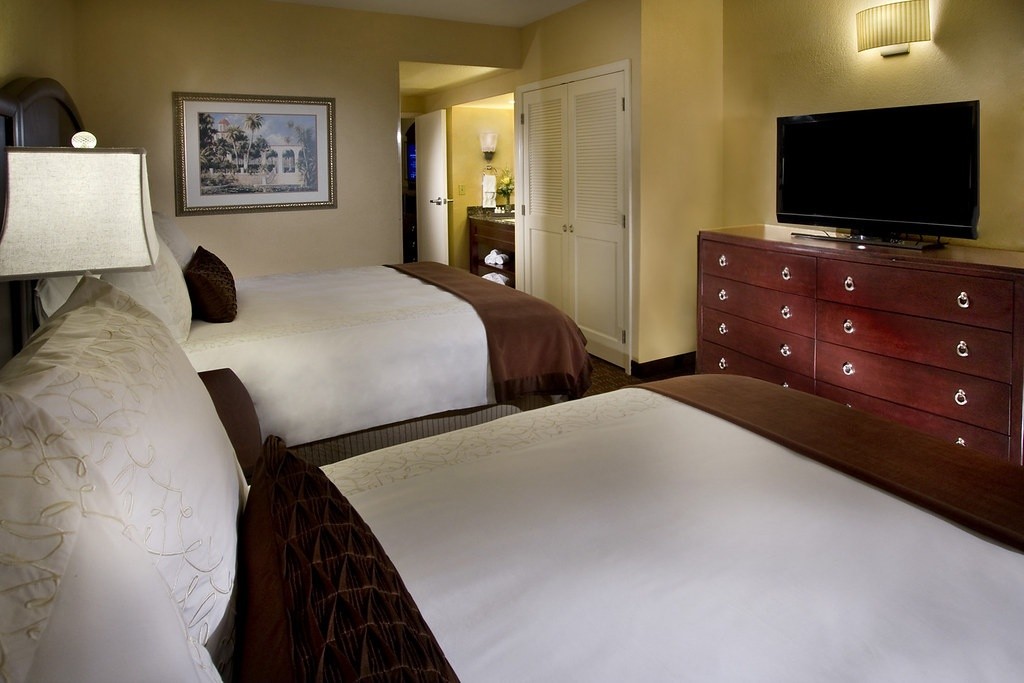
[502,217,515,222]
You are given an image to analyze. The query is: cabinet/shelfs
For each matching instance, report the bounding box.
[518,58,632,377]
[469,220,516,287]
[695,223,1024,469]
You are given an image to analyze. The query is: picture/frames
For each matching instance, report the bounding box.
[173,91,338,216]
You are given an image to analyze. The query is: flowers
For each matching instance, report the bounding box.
[484,165,515,201]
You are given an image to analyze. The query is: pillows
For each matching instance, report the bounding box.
[186,245,237,323]
[34,209,195,346]
[0,271,249,683]
[241,436,461,683]
[0,389,224,683]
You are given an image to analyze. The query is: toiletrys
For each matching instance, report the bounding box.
[495,206,505,213]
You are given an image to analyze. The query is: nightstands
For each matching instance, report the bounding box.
[199,367,263,482]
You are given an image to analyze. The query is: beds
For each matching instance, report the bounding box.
[181,259,596,469]
[320,371,1024,683]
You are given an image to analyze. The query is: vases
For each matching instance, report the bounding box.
[503,196,511,213]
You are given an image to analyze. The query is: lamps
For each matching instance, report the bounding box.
[480,131,499,164]
[856,0,932,57]
[0,131,159,284]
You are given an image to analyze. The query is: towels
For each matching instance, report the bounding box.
[481,273,509,285]
[481,175,497,208]
[484,248,509,265]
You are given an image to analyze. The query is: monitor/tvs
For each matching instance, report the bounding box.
[776,100,981,249]
